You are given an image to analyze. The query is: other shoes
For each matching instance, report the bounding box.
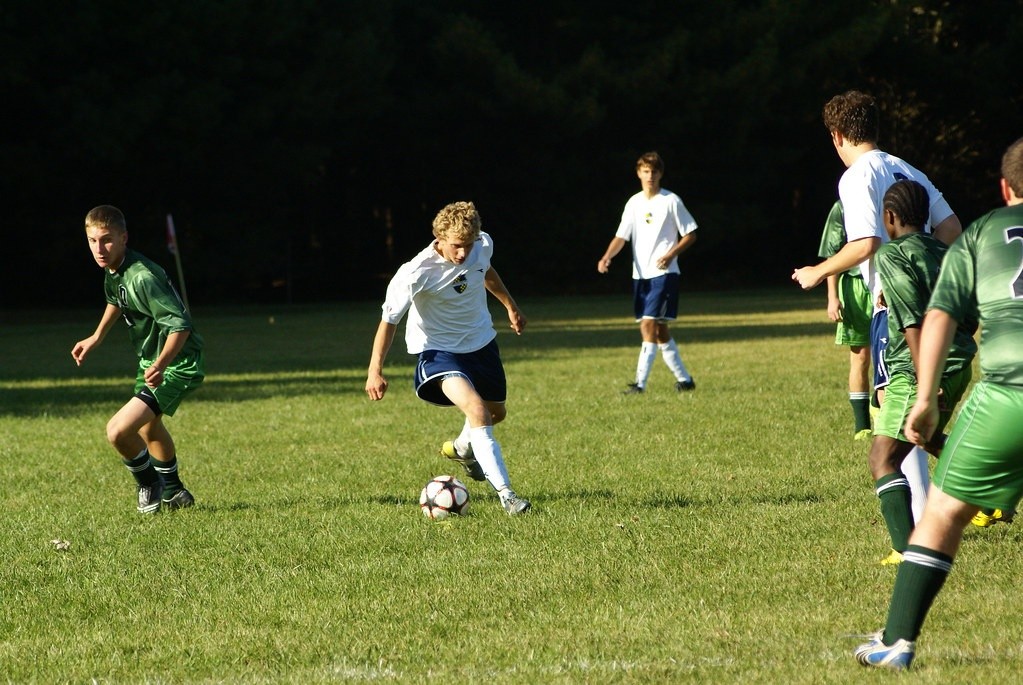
[854,429,873,442]
[673,376,695,393]
[622,383,643,396]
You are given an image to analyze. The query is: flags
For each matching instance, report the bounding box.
[166,213,177,254]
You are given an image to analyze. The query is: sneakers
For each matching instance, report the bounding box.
[880,547,903,566]
[163,488,194,511]
[441,440,486,481]
[848,626,916,673]
[503,492,532,515]
[135,470,165,516]
[971,508,1002,526]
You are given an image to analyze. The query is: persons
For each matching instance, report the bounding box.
[366,201,531,518]
[72,205,205,516]
[792,90,1017,563]
[841,139,1023,672]
[598,152,698,394]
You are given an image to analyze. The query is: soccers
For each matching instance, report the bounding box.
[418,474,471,522]
